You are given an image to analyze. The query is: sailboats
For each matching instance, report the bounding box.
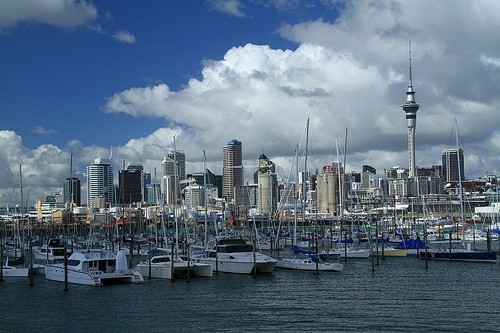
[0,160,46,278]
[189,148,280,276]
[43,154,146,288]
[0,131,500,263]
[135,136,212,281]
[271,117,345,273]
